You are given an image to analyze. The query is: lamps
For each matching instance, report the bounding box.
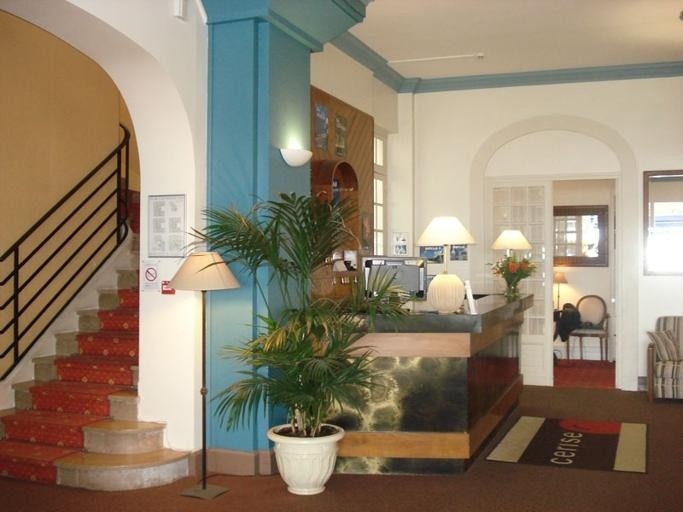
[165,251,243,503]
[491,228,533,251]
[554,271,569,311]
[415,216,477,274]
[276,148,313,170]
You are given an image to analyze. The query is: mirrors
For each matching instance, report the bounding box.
[640,168,682,278]
[553,204,610,268]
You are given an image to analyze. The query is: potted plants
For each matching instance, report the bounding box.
[179,189,413,497]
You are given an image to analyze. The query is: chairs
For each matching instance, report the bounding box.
[566,293,611,362]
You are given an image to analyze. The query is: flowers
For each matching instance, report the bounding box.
[485,251,538,296]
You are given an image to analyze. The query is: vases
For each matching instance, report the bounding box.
[506,283,517,296]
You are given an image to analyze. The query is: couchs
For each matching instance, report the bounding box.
[646,313,683,405]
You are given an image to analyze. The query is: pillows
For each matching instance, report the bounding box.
[647,328,682,362]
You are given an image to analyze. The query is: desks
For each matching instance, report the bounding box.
[308,288,536,472]
